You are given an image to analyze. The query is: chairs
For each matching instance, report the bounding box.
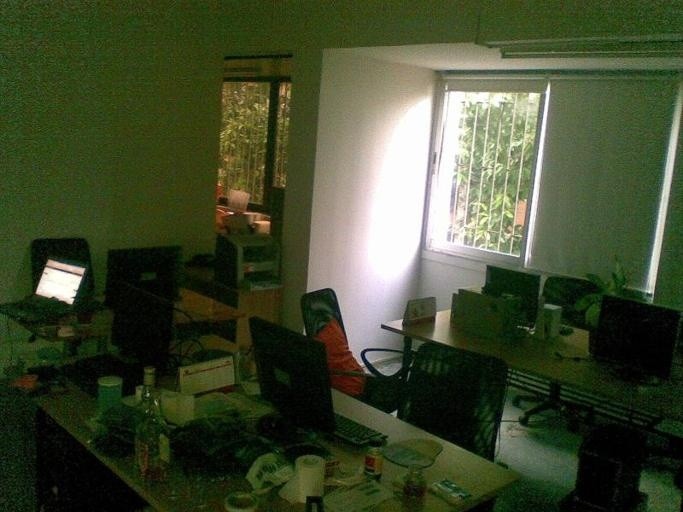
[395,341,508,461]
[513,276,601,433]
[300,288,413,414]
[31,238,96,295]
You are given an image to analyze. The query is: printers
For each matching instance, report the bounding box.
[215,233,280,289]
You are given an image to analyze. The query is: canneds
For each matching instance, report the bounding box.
[364,447,385,475]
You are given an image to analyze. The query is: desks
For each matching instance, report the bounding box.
[26,334,521,512]
[0,286,246,357]
[211,280,285,354]
[381,308,683,424]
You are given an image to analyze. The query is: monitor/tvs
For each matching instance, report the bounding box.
[248,316,337,448]
[485,264,541,338]
[111,282,174,355]
[103,246,182,306]
[592,295,681,386]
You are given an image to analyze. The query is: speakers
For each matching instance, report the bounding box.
[543,303,562,339]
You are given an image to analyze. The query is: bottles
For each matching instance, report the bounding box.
[362,439,385,479]
[403,464,427,500]
[131,366,163,485]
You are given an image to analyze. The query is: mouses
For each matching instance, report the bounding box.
[55,326,81,338]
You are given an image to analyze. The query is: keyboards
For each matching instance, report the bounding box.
[559,324,574,336]
[75,353,143,397]
[332,410,389,448]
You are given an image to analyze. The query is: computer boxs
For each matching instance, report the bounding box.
[576,424,647,510]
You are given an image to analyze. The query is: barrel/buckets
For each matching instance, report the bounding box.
[278,456,326,505]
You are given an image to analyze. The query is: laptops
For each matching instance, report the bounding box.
[0,256,90,323]
